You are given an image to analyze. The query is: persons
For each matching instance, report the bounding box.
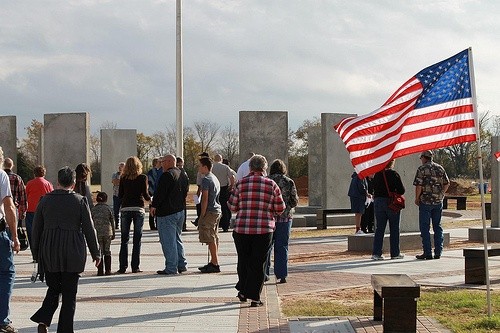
[111,162,126,229]
[348,171,372,234]
[236,152,267,182]
[87,192,116,275]
[147,156,190,231]
[370,158,406,261]
[2,157,27,239]
[0,146,18,333]
[196,158,221,273]
[149,154,189,275]
[19,167,54,249]
[263,158,299,283]
[412,150,450,260]
[190,153,237,233]
[30,166,102,333]
[228,154,286,307]
[115,155,151,274]
[71,165,94,209]
[361,176,374,233]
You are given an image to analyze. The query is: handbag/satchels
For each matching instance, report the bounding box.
[17,229,29,250]
[388,191,405,213]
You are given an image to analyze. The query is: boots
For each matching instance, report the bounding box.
[97,258,104,276]
[104,256,112,274]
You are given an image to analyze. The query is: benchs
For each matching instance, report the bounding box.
[322,208,354,229]
[481,202,491,220]
[372,273,421,333]
[464,246,500,284]
[443,196,468,210]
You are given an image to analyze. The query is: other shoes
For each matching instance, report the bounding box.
[198,262,221,272]
[416,253,433,259]
[37,324,48,333]
[238,292,247,302]
[156,270,167,274]
[356,230,364,234]
[0,325,19,333]
[251,301,263,307]
[373,254,385,261]
[132,269,143,272]
[280,278,286,283]
[434,255,440,258]
[391,252,404,261]
[118,270,125,273]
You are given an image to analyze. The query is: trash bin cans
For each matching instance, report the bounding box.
[479,183,487,195]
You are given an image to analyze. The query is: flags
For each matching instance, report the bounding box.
[333,48,477,180]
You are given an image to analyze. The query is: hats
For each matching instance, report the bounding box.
[197,151,210,156]
[420,149,433,159]
